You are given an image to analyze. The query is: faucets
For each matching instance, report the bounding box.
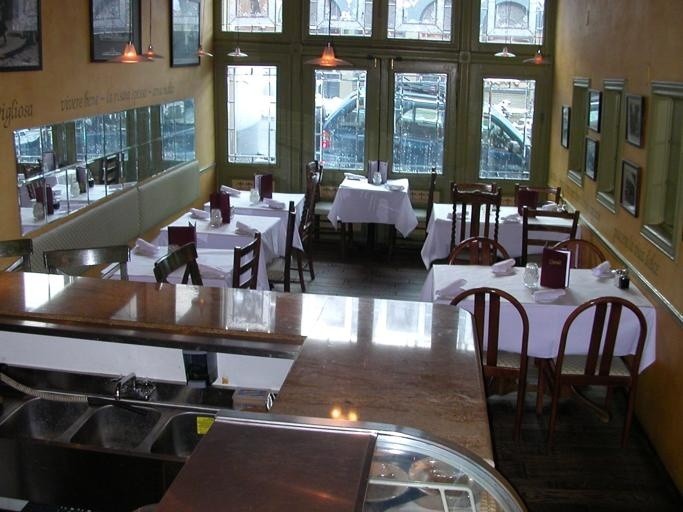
[115,373,137,402]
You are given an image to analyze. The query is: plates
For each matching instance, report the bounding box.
[434,287,466,300]
[233,227,259,235]
[592,267,616,278]
[487,267,519,276]
[528,289,560,303]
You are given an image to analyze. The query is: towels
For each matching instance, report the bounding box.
[446,210,469,220]
[500,211,519,221]
[436,278,468,296]
[220,184,241,197]
[384,181,406,191]
[542,202,558,212]
[235,220,258,237]
[591,260,610,278]
[491,257,516,273]
[533,287,567,304]
[262,197,286,209]
[134,237,156,257]
[344,172,367,181]
[189,205,208,219]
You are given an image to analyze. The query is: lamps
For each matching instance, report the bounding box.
[226,1,249,57]
[523,0,552,65]
[195,0,214,57]
[142,0,165,61]
[304,0,354,67]
[108,0,154,64]
[494,0,516,58]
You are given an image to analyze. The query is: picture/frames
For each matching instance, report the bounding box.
[625,94,647,149]
[0,0,43,72]
[169,0,202,68]
[560,105,571,149]
[584,136,600,181]
[88,0,142,64]
[619,159,642,218]
[586,89,603,133]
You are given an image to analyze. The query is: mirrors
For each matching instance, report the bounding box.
[566,77,591,190]
[595,78,628,216]
[640,80,683,262]
[13,96,196,237]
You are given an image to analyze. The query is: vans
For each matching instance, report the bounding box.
[395,73,439,95]
[320,88,532,178]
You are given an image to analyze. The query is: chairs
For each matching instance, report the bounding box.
[514,183,562,207]
[522,205,581,268]
[412,166,437,240]
[306,160,353,242]
[451,183,503,264]
[450,181,496,193]
[536,297,647,443]
[268,201,297,292]
[0,239,34,272]
[43,245,131,281]
[447,236,511,266]
[450,287,529,442]
[552,239,606,269]
[153,241,204,286]
[99,152,125,185]
[290,164,323,293]
[26,177,50,200]
[233,232,261,290]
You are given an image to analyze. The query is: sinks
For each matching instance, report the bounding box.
[65,398,163,453]
[148,407,218,460]
[2,390,95,444]
